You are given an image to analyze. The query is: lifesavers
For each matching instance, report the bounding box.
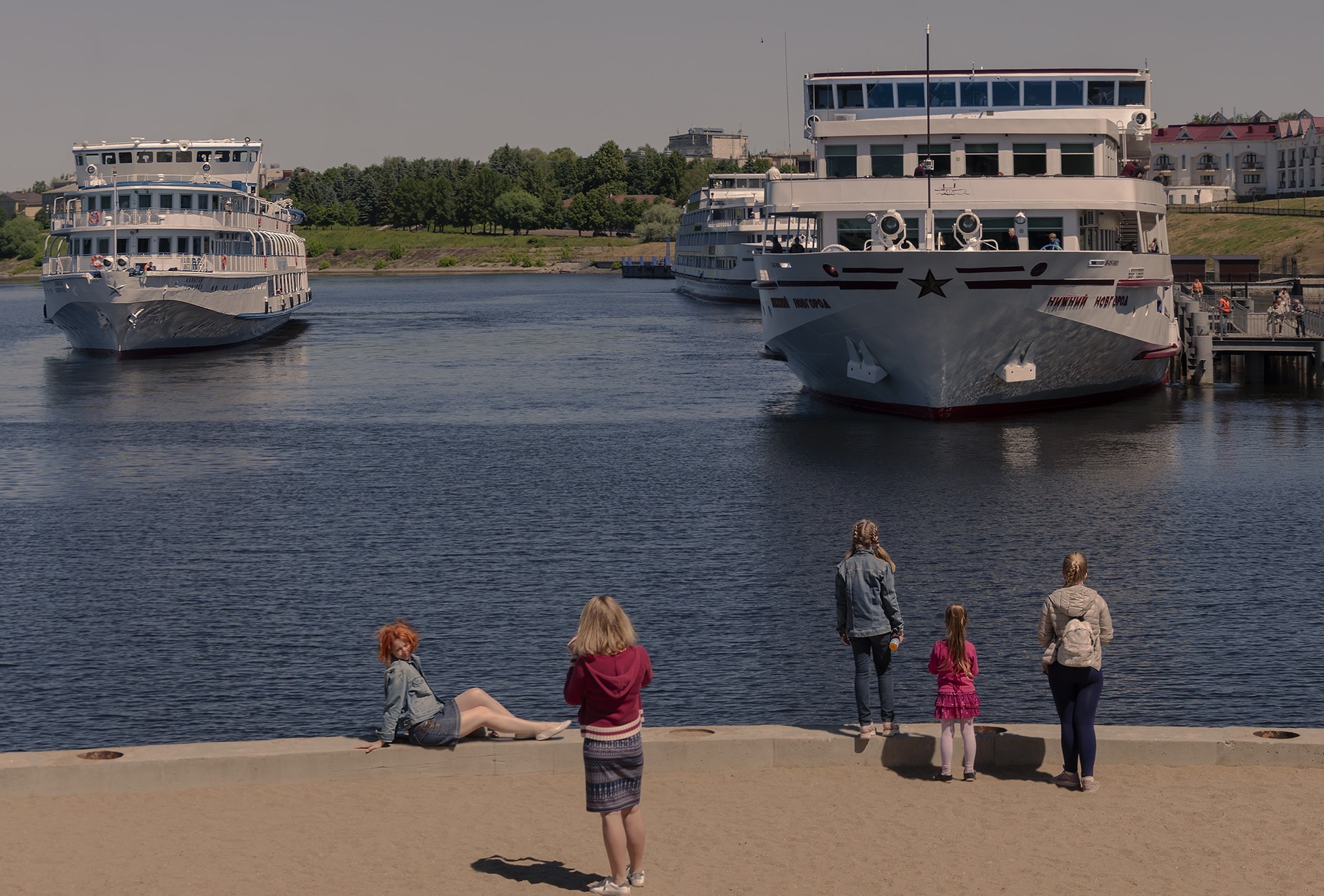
[305,257,307,265]
[222,255,226,266]
[91,255,103,268]
[295,257,297,266]
[277,220,278,229]
[265,258,267,267]
[259,217,262,226]
[286,224,288,231]
[89,211,100,223]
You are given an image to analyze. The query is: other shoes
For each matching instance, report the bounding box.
[536,720,571,740]
[515,733,535,739]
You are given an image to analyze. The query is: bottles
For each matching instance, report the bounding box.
[889,638,900,650]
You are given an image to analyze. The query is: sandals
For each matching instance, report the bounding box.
[860,722,877,739]
[882,722,900,736]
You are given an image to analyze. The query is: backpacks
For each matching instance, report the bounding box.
[1057,616,1096,667]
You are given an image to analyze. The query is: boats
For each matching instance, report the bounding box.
[670,169,818,308]
[737,22,1183,427]
[40,137,313,362]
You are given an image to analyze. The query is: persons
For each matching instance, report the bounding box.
[834,519,904,739]
[1148,237,1159,253]
[1153,173,1164,183]
[563,595,653,896]
[789,237,805,253]
[770,236,783,253]
[914,161,926,178]
[1192,279,1203,297]
[766,161,782,181]
[1120,161,1151,179]
[928,603,979,783]
[1157,300,1162,313]
[144,262,153,271]
[1007,228,1019,250]
[1266,287,1306,337]
[1215,294,1233,336]
[1037,552,1113,793]
[354,622,572,753]
[1049,233,1060,250]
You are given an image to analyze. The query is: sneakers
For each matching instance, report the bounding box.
[963,770,976,781]
[1049,770,1080,787]
[626,865,645,887]
[589,876,630,896]
[1081,777,1099,791]
[932,769,953,783]
